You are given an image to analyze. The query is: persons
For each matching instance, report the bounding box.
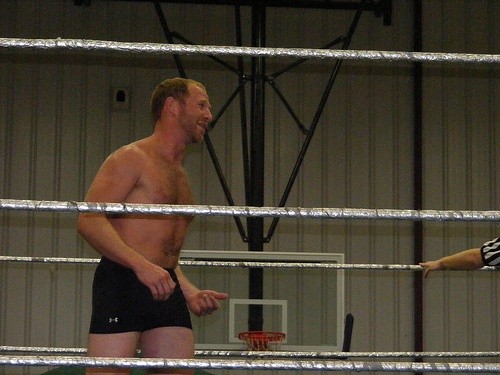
[419,235,500,276]
[73,77,228,375]
